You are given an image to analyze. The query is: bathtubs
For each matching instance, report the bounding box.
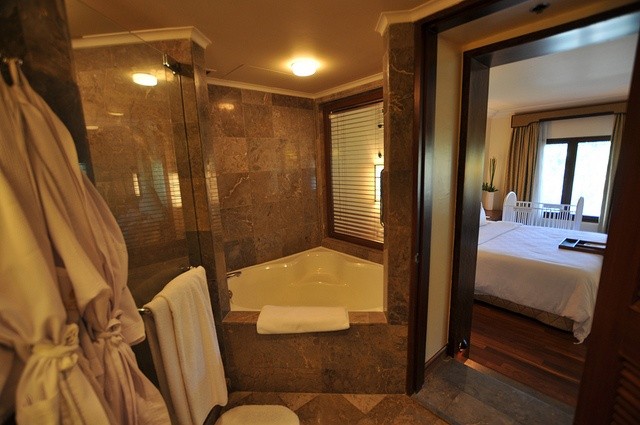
[228,248,386,315]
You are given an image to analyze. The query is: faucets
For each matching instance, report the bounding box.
[226,269,242,280]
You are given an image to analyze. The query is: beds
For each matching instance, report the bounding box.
[475,205,608,344]
[502,191,584,231]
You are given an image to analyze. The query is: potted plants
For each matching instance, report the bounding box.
[482,155,497,210]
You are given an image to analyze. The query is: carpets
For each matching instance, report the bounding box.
[213,405,300,424]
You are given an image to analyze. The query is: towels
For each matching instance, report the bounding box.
[255,303,350,336]
[142,265,230,423]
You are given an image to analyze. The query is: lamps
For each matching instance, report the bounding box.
[132,72,158,87]
[289,58,319,76]
